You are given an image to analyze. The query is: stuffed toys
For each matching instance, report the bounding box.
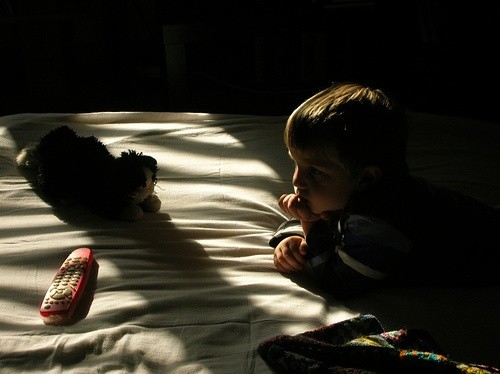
[13,124,162,223]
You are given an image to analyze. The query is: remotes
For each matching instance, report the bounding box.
[40,247,93,325]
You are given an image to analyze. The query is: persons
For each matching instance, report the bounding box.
[268,81,500,303]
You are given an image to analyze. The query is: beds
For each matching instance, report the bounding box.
[0,111,499,373]
